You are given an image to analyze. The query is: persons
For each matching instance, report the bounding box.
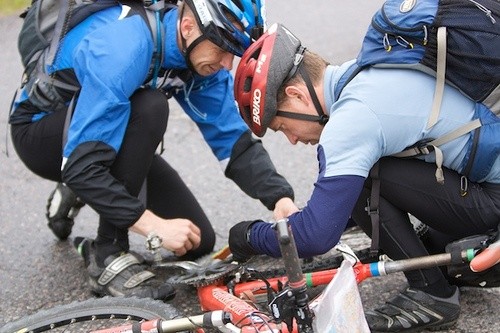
[10,0,298,303]
[228,0,500,333]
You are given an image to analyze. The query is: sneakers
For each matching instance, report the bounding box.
[45,182,85,242]
[86,252,178,304]
[364,286,462,333]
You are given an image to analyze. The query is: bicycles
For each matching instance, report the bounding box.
[1,216,500,333]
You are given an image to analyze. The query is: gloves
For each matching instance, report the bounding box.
[228,219,263,262]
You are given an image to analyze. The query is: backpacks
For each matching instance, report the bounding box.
[17,0,179,90]
[331,0,499,125]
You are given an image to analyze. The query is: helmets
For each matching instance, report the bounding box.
[235,22,301,137]
[185,0,266,57]
